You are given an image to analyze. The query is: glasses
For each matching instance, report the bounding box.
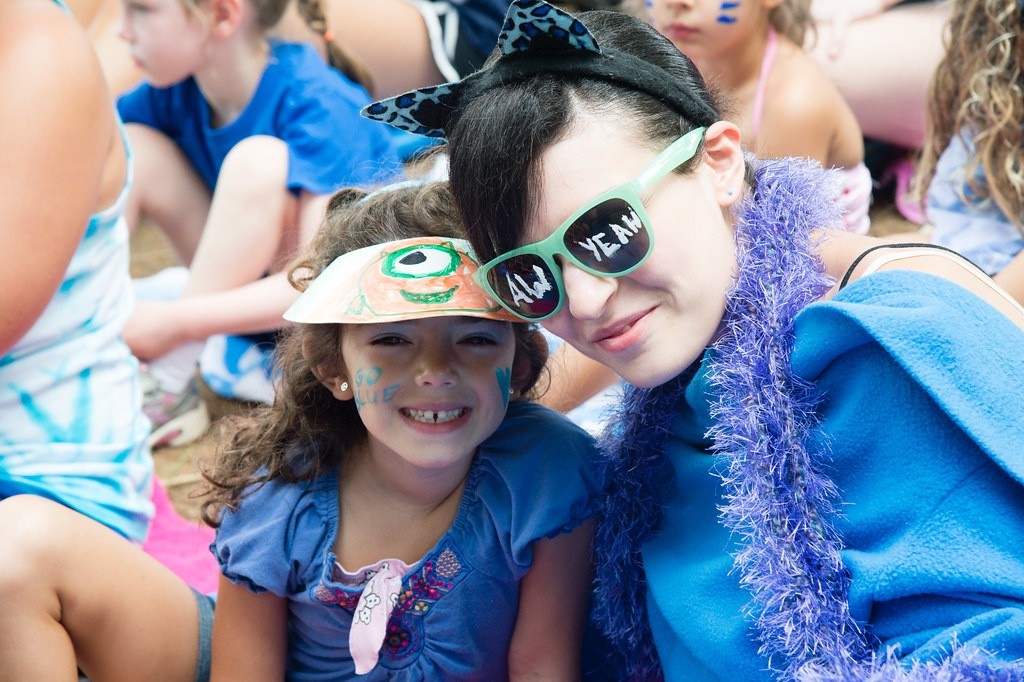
[471,125,707,323]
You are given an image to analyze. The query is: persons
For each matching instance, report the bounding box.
[212,185,605,682]
[450,12,1024,682]
[0,0,1024,682]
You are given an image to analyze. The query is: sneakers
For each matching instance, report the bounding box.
[138,361,210,454]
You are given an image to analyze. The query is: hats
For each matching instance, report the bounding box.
[281,236,528,323]
